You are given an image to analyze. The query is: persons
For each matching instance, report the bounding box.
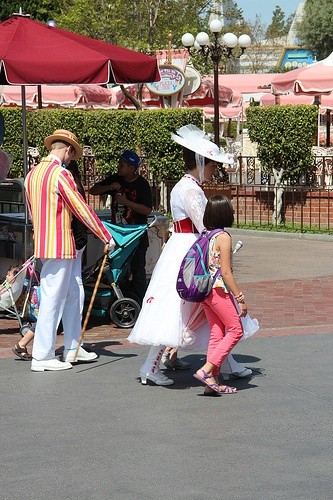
[0,128,117,371]
[89,150,152,302]
[127,124,253,396]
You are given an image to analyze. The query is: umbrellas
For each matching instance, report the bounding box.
[0,52,333,147]
[0,7,160,263]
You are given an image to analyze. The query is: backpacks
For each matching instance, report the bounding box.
[176,229,223,303]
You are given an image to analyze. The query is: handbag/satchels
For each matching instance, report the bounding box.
[22,259,40,323]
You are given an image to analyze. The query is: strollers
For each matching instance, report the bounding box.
[0,255,37,337]
[56,214,156,335]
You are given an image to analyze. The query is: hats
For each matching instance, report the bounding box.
[43,129,83,160]
[171,124,237,165]
[116,150,140,165]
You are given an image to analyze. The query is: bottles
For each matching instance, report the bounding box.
[116,188,124,208]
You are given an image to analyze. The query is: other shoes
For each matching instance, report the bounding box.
[162,352,192,369]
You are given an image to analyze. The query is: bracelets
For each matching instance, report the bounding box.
[235,292,245,302]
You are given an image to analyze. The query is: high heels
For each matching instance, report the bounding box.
[222,368,252,380]
[139,372,174,385]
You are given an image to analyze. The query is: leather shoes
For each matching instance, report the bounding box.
[30,358,73,371]
[63,346,98,363]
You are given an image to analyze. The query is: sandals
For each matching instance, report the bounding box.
[204,384,238,394]
[193,367,220,394]
[12,341,32,360]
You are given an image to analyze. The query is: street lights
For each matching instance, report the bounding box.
[183,20,251,148]
